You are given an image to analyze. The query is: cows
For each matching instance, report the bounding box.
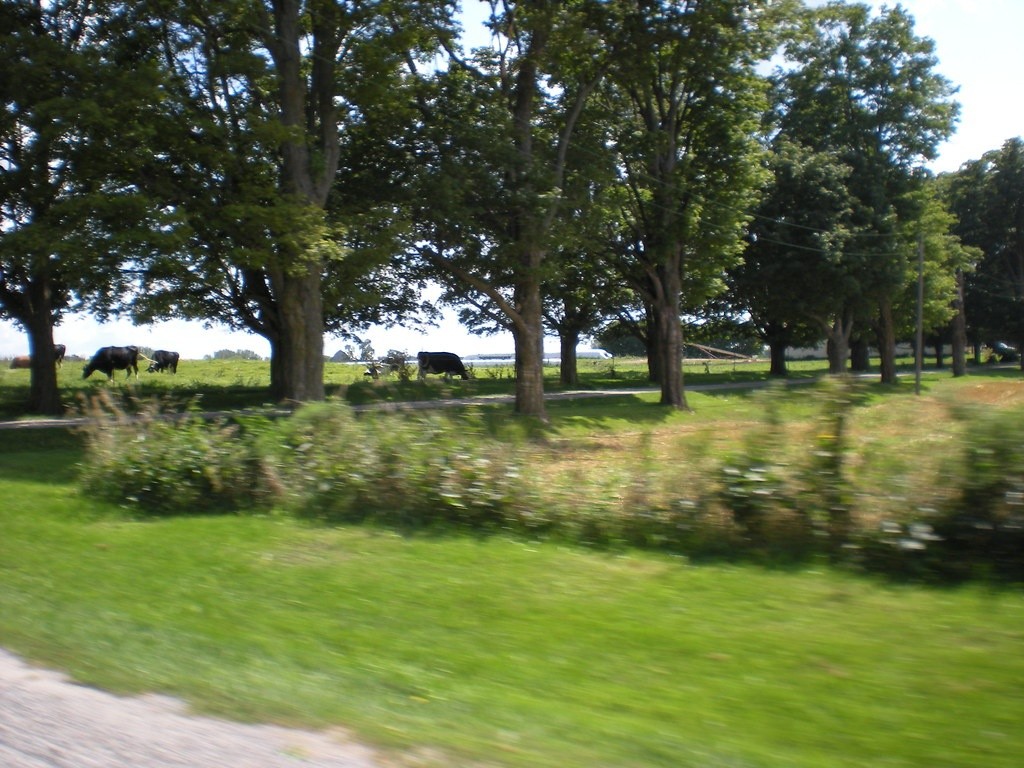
[145,349,180,375]
[418,351,469,380]
[81,344,139,382]
[10,343,66,371]
